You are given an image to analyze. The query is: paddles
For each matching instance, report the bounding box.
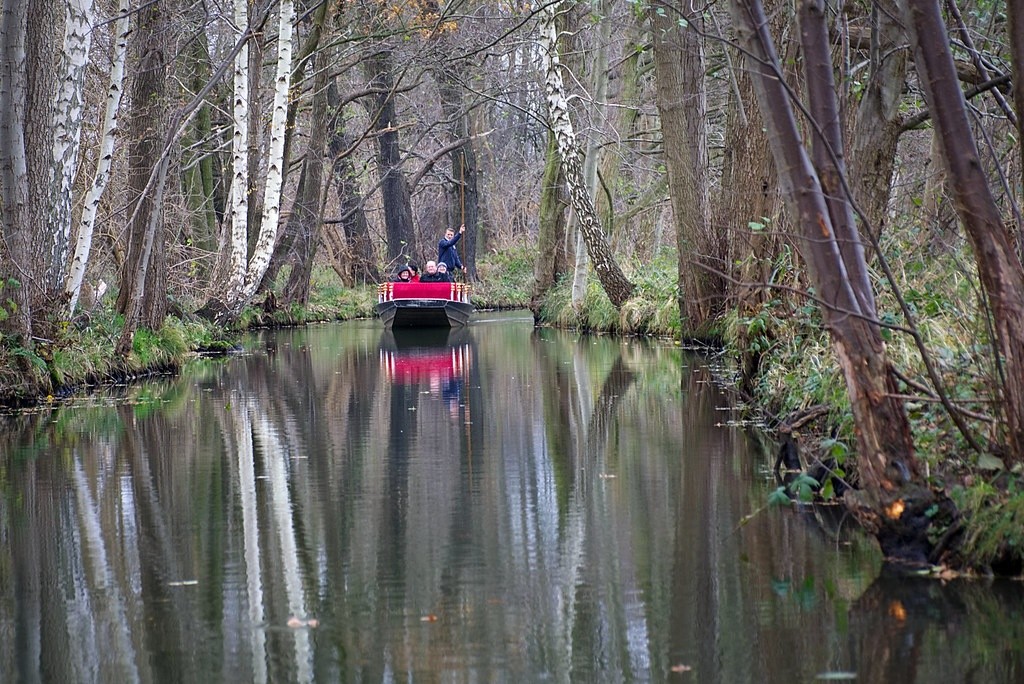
[460,151,466,283]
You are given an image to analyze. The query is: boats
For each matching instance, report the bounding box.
[376,297,471,332]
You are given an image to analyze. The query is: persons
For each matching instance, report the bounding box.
[439,223,466,281]
[419,261,449,282]
[407,266,420,282]
[394,265,411,282]
[437,262,453,282]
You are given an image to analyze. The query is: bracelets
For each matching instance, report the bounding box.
[462,266,466,270]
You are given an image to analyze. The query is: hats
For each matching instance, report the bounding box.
[397,266,411,277]
[436,262,447,270]
[408,265,417,272]
